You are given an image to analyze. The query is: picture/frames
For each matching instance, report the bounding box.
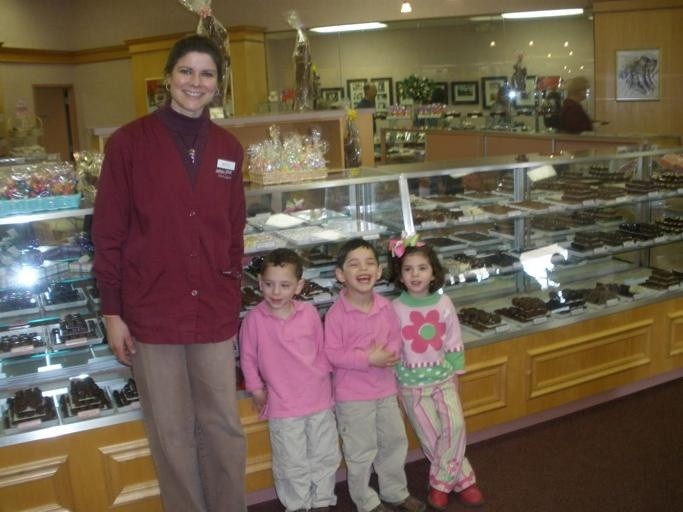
[451,81,479,104]
[345,78,367,109]
[395,81,416,108]
[421,80,448,107]
[319,87,343,104]
[370,77,393,109]
[511,74,539,109]
[612,46,662,103]
[481,77,507,110]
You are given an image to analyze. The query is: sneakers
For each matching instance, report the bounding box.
[458,483,483,507]
[382,494,426,512]
[427,484,449,510]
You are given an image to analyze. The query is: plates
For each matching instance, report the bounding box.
[245,207,390,255]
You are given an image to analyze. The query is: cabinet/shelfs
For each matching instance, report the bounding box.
[0,147,681,451]
[377,122,681,170]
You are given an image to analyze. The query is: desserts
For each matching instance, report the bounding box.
[1,164,683,430]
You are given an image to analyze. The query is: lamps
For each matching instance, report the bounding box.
[398,1,413,14]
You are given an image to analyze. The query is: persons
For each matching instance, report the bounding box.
[238,247,341,511]
[386,234,485,508]
[90,35,256,512]
[324,238,428,511]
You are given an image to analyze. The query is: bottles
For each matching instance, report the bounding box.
[289,43,322,109]
